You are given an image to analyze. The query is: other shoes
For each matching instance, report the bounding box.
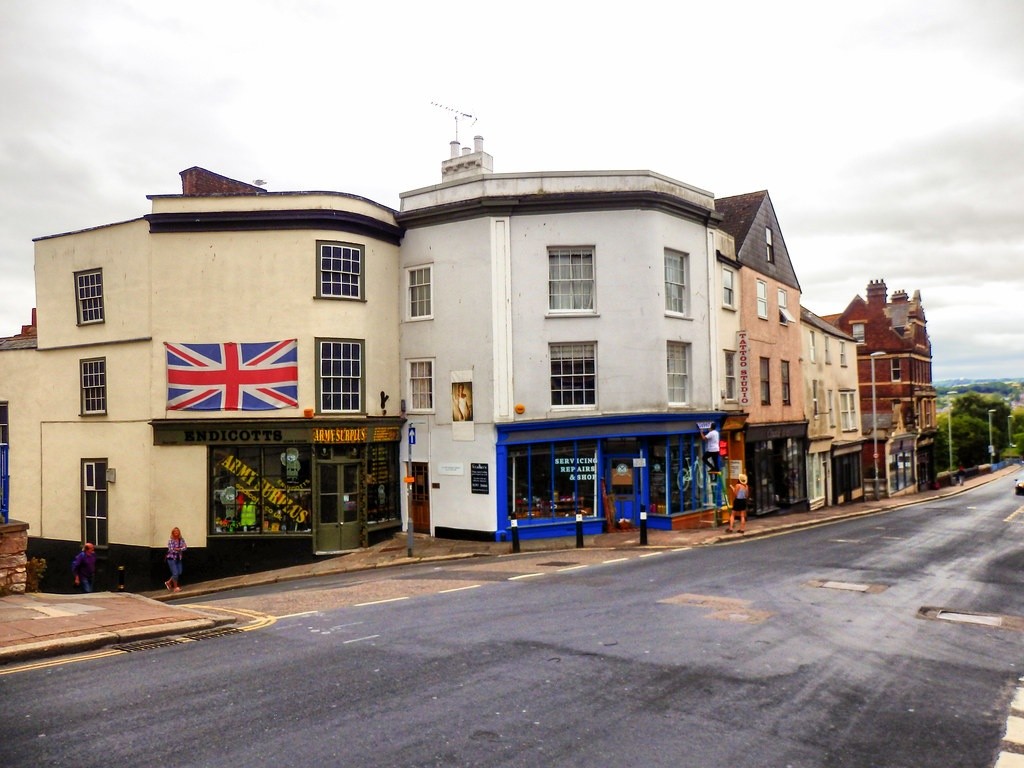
[165,581,171,591]
[726,528,732,532]
[736,529,744,533]
[708,468,720,472]
[174,587,183,592]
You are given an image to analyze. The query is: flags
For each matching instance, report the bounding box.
[162,339,300,412]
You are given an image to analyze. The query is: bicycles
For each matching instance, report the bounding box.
[677,456,704,491]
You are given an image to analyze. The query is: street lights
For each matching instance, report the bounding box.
[1007,413,1012,462]
[870,349,885,500]
[947,389,958,485]
[987,407,997,468]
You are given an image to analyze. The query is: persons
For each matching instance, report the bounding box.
[958,466,966,486]
[699,423,720,473]
[72,542,96,593]
[724,473,748,534]
[164,526,187,593]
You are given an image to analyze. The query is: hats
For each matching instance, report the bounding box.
[738,474,748,484]
